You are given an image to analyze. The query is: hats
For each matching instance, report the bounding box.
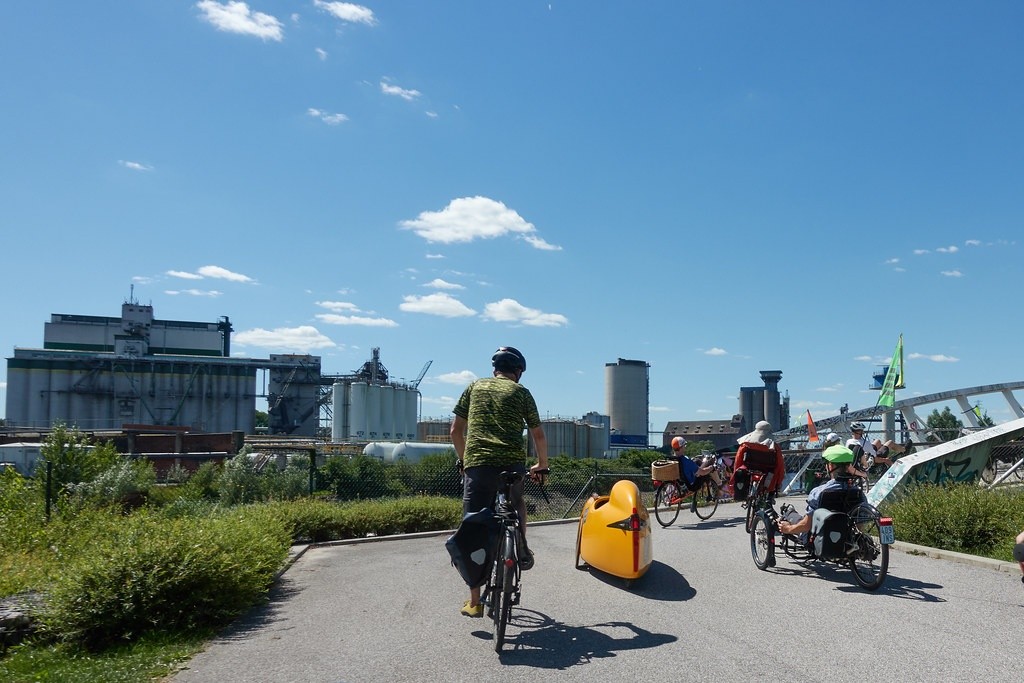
[827,433,842,443]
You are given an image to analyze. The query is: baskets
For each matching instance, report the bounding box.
[652,460,680,481]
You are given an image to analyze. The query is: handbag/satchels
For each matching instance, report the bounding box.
[445,507,497,587]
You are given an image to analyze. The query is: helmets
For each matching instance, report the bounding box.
[671,436,687,450]
[821,446,854,464]
[849,423,866,432]
[491,346,526,373]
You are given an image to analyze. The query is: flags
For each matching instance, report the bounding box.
[877,336,906,408]
[807,410,819,443]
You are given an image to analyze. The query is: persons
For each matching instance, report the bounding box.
[1015,531,1024,584]
[847,421,913,472]
[450,347,549,618]
[670,437,729,493]
[733,420,785,506]
[778,447,869,547]
[826,432,866,479]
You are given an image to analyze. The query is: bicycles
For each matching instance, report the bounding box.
[575,480,652,589]
[652,452,723,527]
[750,490,896,591]
[814,452,1024,494]
[741,471,781,533]
[456,458,551,652]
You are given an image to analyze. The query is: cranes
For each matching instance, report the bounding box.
[412,360,433,389]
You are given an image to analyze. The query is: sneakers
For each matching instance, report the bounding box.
[518,542,534,569]
[460,599,483,618]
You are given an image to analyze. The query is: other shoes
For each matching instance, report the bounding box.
[718,479,729,490]
